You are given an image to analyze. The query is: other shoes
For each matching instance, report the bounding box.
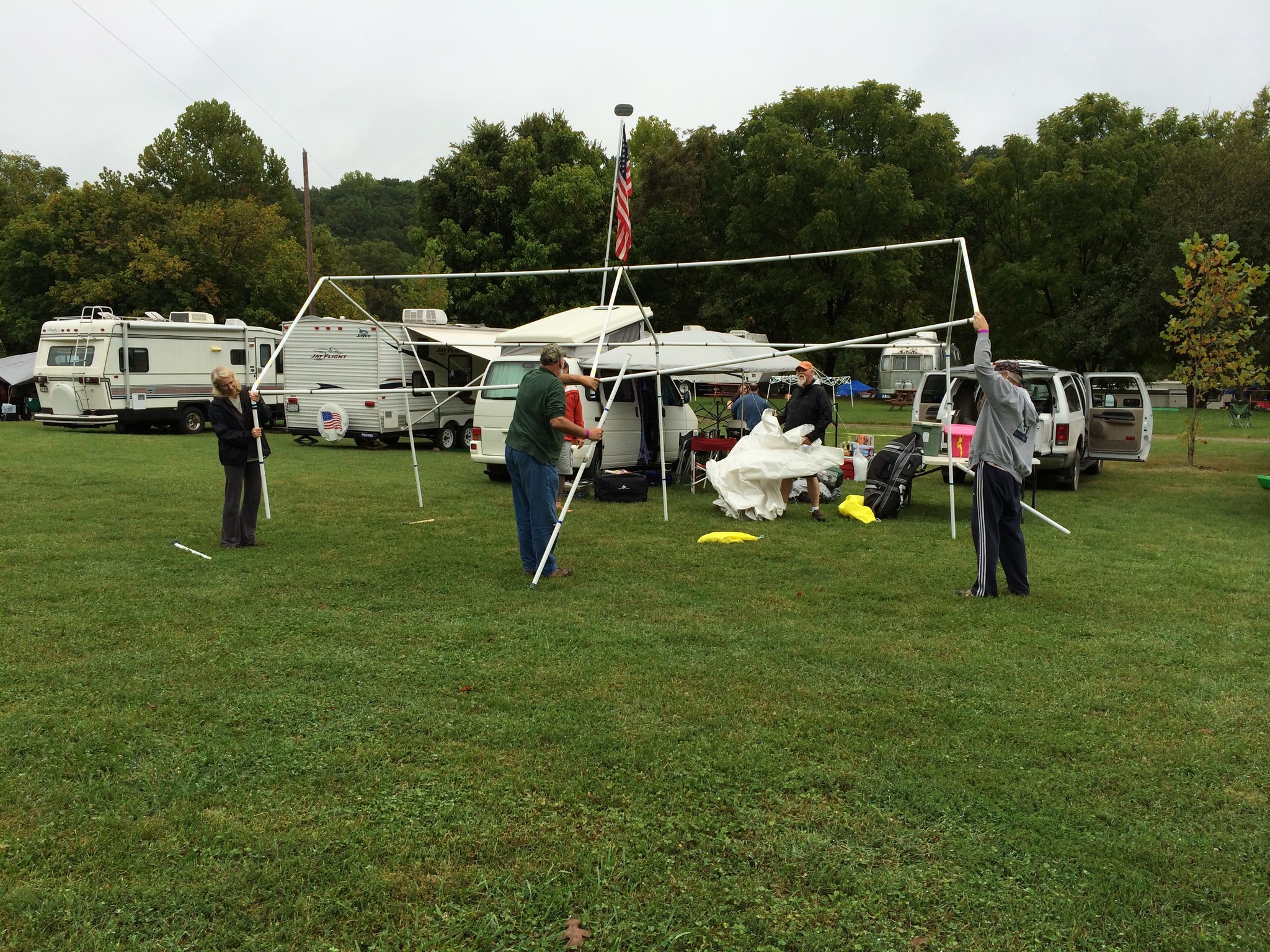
[525,570,536,577]
[546,567,572,578]
[955,589,975,597]
[997,587,1012,595]
[777,509,787,517]
[811,509,826,522]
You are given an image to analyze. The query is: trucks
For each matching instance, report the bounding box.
[34,306,284,434]
[644,326,779,395]
[470,305,699,482]
[279,309,512,451]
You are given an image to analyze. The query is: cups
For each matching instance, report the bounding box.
[713,429,720,439]
[701,431,709,438]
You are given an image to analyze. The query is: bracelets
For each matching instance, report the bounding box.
[977,329,989,334]
[585,429,590,439]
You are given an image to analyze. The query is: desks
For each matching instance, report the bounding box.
[907,456,1041,524]
[691,437,736,494]
[890,389,917,411]
[706,383,741,405]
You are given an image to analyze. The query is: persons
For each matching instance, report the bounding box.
[772,361,833,522]
[731,382,775,432]
[555,360,585,511]
[209,366,272,548]
[727,383,748,420]
[505,343,604,580]
[956,312,1039,598]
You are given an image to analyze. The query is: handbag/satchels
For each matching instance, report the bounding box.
[594,470,648,503]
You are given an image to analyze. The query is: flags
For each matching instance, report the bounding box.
[320,411,342,432]
[615,123,632,263]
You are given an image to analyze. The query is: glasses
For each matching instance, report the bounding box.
[798,369,808,373]
[220,378,235,390]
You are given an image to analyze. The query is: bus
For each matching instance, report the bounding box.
[879,331,962,398]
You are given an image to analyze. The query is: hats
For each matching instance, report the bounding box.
[994,361,1023,377]
[795,361,813,369]
[540,343,567,362]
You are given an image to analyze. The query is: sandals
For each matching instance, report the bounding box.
[555,506,573,513]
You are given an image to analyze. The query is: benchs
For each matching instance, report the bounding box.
[885,398,914,411]
[703,392,737,405]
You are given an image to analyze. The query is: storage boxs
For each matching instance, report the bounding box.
[911,421,944,456]
[945,424,976,458]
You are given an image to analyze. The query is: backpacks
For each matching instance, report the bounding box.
[816,467,844,487]
[864,432,924,519]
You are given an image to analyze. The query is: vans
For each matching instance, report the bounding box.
[911,359,1153,490]
